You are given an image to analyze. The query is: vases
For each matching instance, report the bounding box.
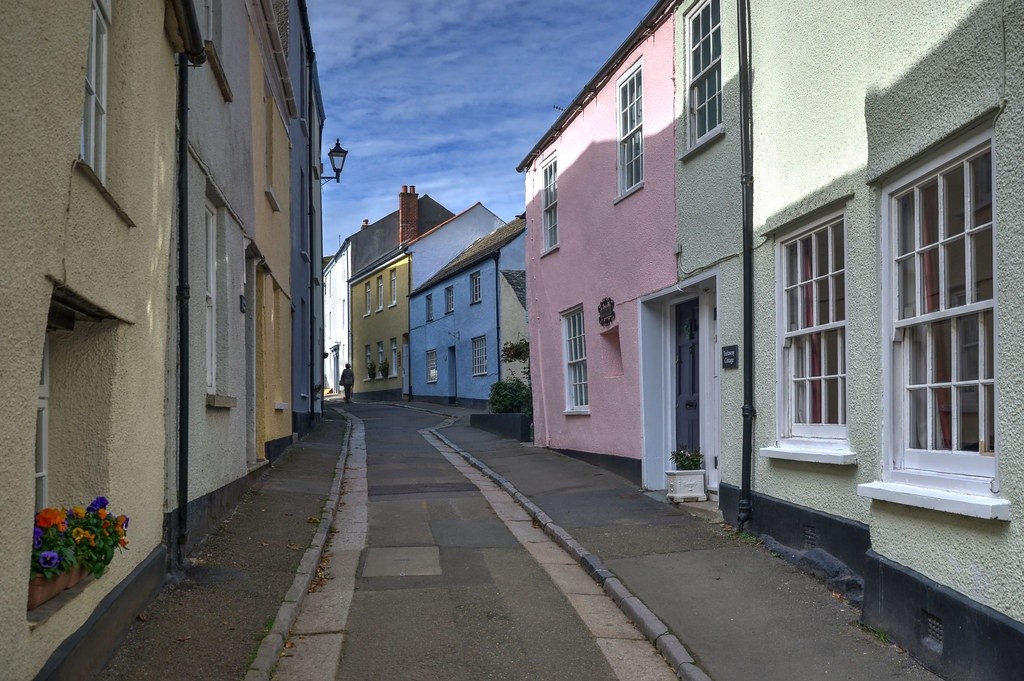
[28,563,87,611]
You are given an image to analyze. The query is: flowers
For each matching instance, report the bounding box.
[30,488,130,580]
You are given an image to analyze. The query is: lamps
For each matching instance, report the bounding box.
[318,136,348,183]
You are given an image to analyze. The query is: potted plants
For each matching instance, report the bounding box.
[665,443,707,503]
[366,359,377,379]
[377,358,389,378]
[500,340,530,364]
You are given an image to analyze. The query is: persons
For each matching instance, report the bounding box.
[341,363,354,403]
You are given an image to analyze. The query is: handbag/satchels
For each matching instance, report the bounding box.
[340,379,345,386]
